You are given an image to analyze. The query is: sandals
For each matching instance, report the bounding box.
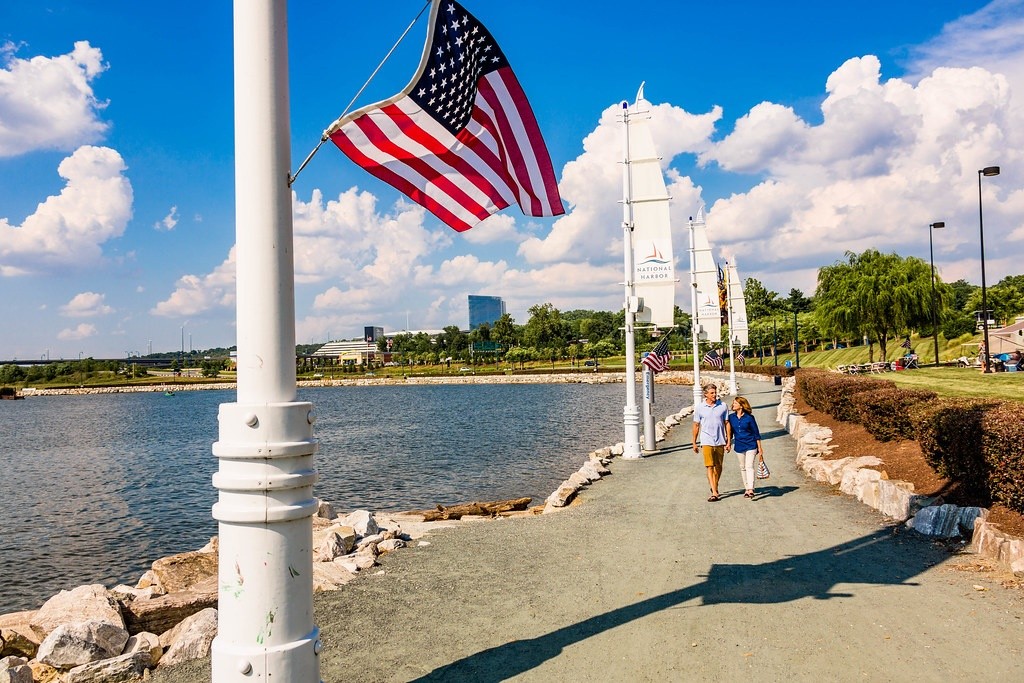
[708,488,722,502]
[744,493,755,499]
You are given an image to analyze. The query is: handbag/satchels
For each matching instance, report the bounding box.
[756,455,770,479]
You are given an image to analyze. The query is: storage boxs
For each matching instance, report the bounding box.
[891,362,904,371]
[1005,365,1017,372]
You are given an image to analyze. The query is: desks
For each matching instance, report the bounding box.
[904,356,919,371]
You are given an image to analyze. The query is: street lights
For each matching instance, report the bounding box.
[930,222,944,366]
[978,166,1001,372]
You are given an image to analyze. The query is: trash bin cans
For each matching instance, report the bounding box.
[785,360,792,367]
[774,375,781,385]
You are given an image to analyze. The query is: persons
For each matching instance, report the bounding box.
[726,397,763,499]
[692,384,731,502]
[1014,351,1024,371]
[978,340,986,372]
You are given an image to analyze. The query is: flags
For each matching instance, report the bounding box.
[900,337,910,348]
[737,350,745,364]
[368,337,372,341]
[324,0,573,233]
[703,350,723,366]
[643,339,671,372]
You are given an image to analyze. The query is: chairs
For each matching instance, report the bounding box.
[832,362,889,376]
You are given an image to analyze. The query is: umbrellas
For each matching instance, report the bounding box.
[993,353,1011,362]
